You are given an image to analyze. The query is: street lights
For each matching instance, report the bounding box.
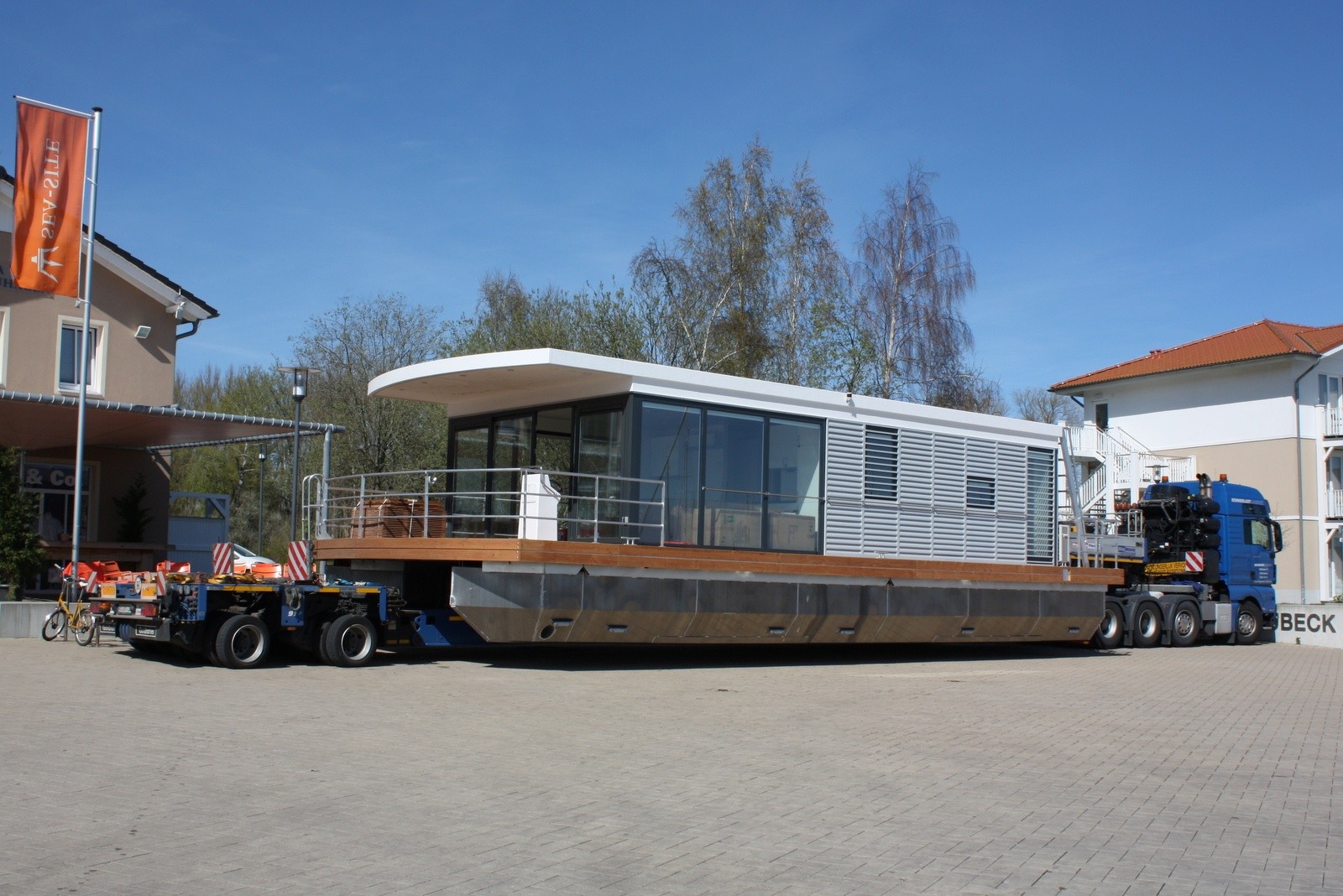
[276,366,323,541]
[251,441,271,555]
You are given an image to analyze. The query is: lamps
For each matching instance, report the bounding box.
[134,325,151,339]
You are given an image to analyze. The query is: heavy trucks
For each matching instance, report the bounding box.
[95,469,1283,669]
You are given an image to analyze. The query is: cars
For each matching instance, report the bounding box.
[231,543,275,573]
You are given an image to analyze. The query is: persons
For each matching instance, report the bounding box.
[31,504,62,591]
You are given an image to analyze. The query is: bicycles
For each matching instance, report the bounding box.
[41,564,97,646]
